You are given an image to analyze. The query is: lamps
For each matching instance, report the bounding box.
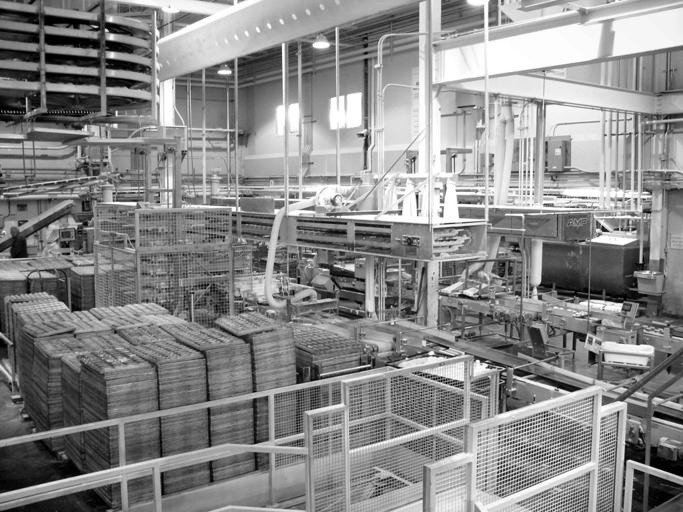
[217,64,232,75]
[312,34,330,49]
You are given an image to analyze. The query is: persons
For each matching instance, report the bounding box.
[8,226,28,259]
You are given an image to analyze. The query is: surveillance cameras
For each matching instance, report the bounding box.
[358,129,368,138]
